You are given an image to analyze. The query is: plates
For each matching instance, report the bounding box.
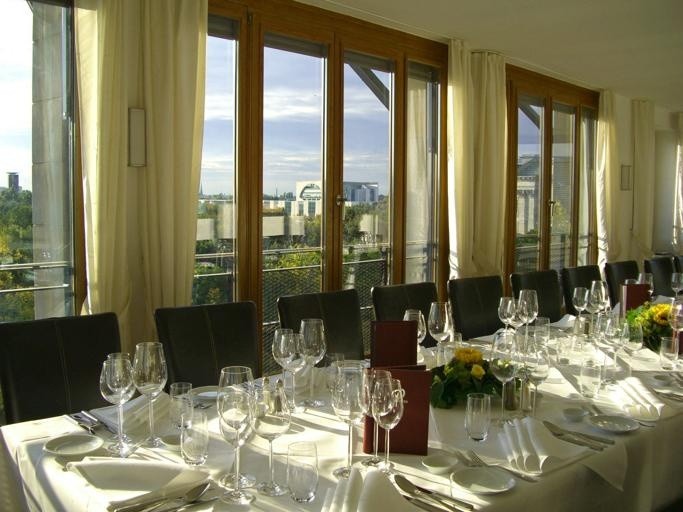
[188,385,219,401]
[40,434,103,460]
[587,415,640,435]
[452,465,517,493]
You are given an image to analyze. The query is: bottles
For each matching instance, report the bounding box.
[261,377,290,418]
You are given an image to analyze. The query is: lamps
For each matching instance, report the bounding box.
[621,164,632,190]
[129,107,148,167]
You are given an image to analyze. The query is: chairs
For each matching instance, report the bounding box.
[0,312,121,423]
[510,269,561,323]
[604,260,640,309]
[560,265,601,316]
[277,289,364,367]
[448,275,505,340]
[371,281,440,347]
[644,256,673,297]
[154,301,261,393]
[675,255,683,273]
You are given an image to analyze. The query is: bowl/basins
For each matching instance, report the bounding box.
[421,455,458,474]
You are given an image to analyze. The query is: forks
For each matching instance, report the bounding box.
[457,449,537,483]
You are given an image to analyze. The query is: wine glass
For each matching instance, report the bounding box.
[490,272,683,427]
[99,342,167,458]
[271,318,404,480]
[215,366,291,507]
[402,301,452,366]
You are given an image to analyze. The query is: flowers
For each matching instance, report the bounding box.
[625,302,675,350]
[424,346,518,408]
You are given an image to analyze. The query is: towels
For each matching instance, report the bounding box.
[607,375,672,420]
[503,424,524,474]
[330,477,348,512]
[276,365,329,400]
[340,467,364,512]
[66,461,185,494]
[553,422,628,491]
[497,432,515,472]
[513,418,542,478]
[356,470,428,512]
[320,487,334,512]
[526,416,591,473]
[91,391,175,434]
[81,455,207,487]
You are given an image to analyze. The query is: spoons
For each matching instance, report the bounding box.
[115,481,210,512]
[542,420,616,447]
[394,473,461,512]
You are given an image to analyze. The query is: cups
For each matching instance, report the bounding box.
[169,380,209,467]
[286,441,319,502]
[466,392,491,443]
[436,333,462,367]
